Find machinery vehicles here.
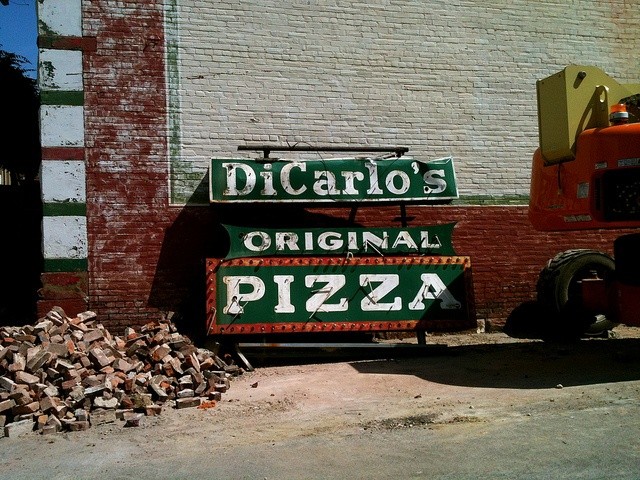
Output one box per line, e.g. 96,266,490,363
530,64,639,338
